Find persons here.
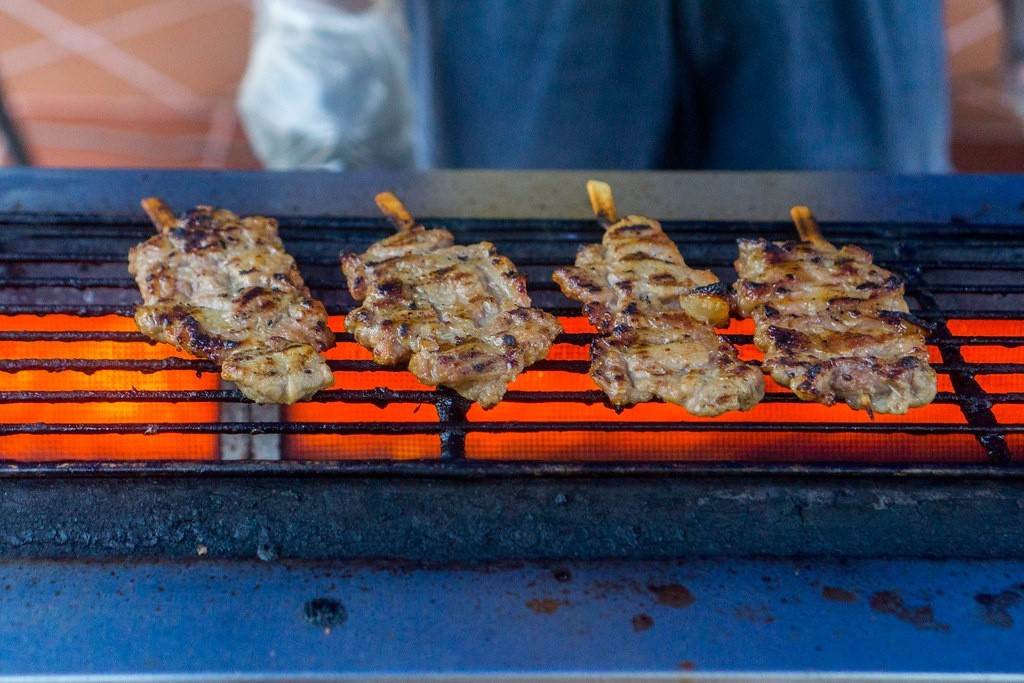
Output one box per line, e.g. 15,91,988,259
310,0,955,177
